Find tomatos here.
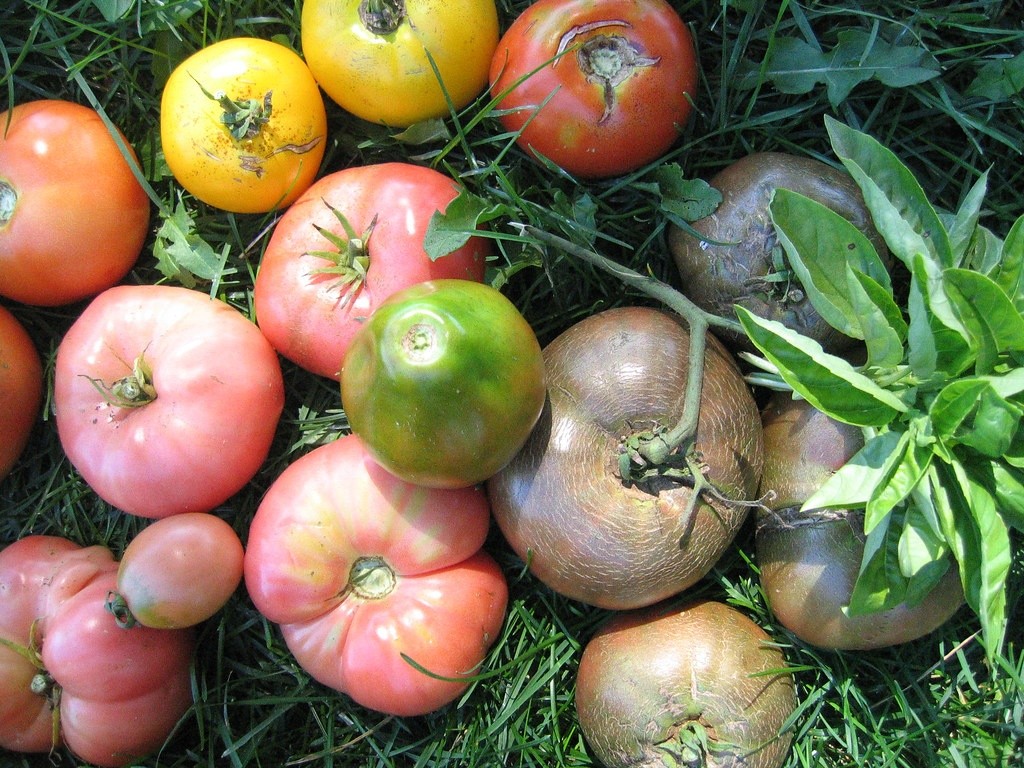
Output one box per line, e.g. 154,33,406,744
0,0,969,768
